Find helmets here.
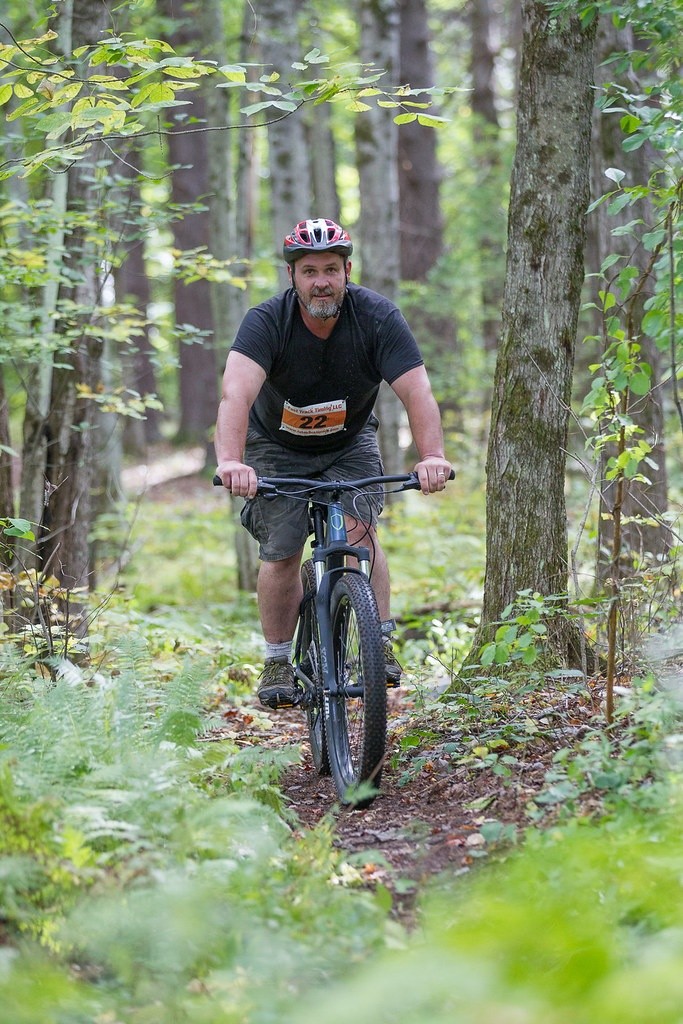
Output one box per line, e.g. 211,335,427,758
283,218,353,262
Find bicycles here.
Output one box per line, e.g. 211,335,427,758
211,463,457,809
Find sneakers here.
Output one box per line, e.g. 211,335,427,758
383,641,404,682
258,654,296,705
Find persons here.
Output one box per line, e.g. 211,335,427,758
212,218,451,706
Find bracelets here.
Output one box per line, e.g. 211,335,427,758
421,452,444,460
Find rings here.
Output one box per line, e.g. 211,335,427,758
438,473,444,475
